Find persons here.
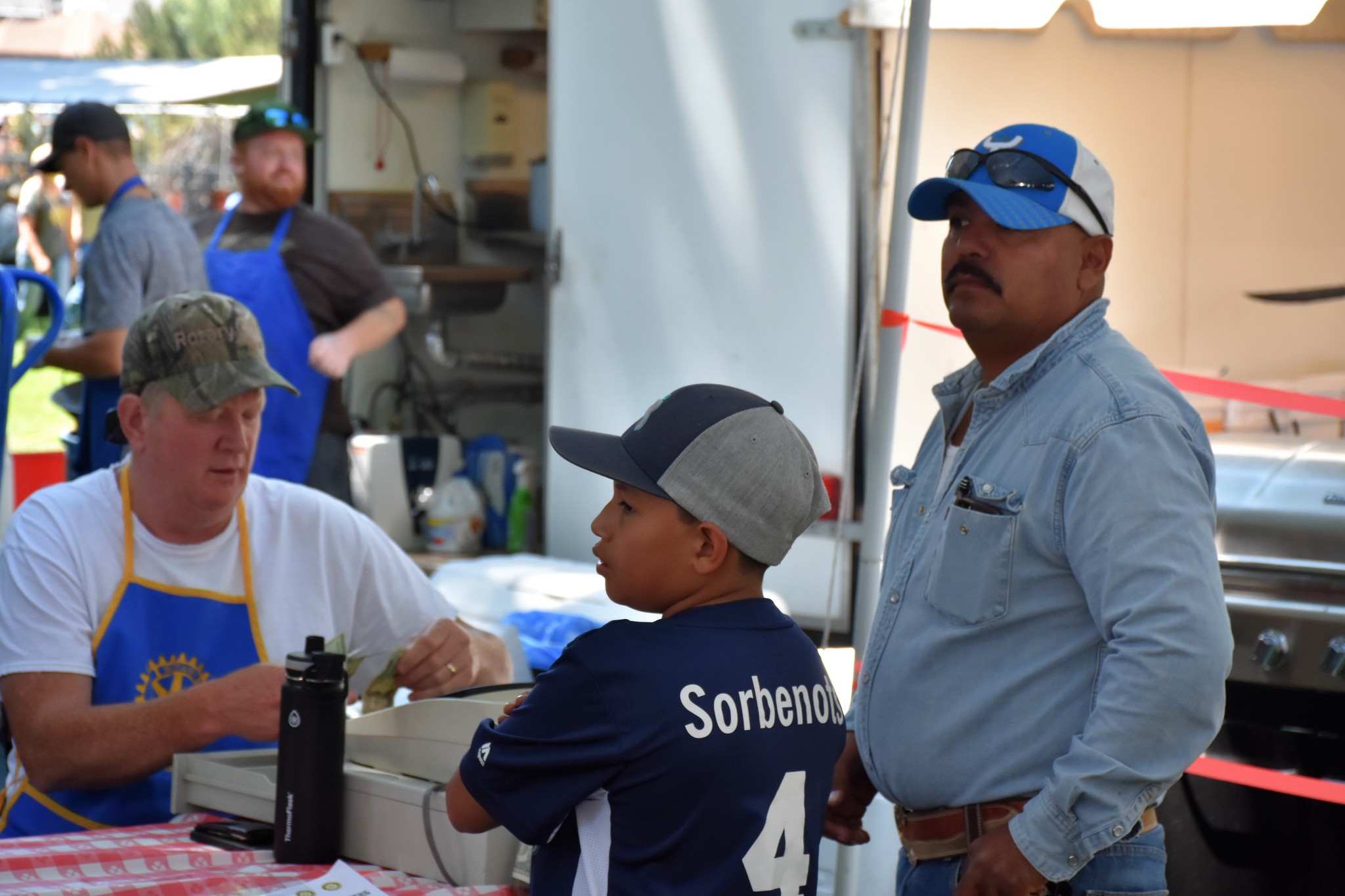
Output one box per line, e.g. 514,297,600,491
446,385,848,896
201,108,407,509
0,286,515,838
821,125,1236,896
31,100,212,479
14,171,82,333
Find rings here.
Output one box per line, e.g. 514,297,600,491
447,663,457,674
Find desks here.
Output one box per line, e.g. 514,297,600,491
0,812,529,896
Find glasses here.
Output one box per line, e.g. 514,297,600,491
233,108,309,130
946,148,1112,237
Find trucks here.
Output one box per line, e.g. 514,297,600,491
542,2,1345,848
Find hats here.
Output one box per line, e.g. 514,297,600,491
29,104,129,174
233,101,317,146
908,122,1114,239
549,383,831,566
121,292,298,414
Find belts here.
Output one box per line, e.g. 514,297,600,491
893,797,1159,865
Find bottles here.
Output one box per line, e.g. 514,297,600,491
272,636,347,862
424,434,534,554
